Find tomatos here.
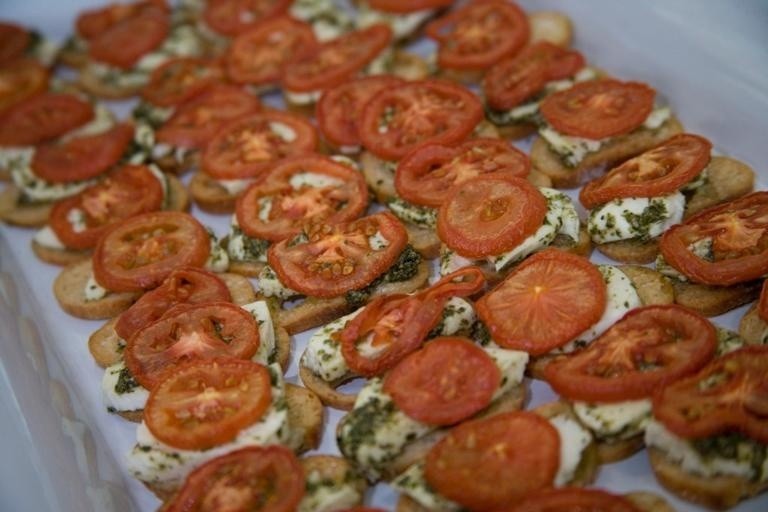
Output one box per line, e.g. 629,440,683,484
74,2,637,510
0,24,305,510
205,1,767,446
